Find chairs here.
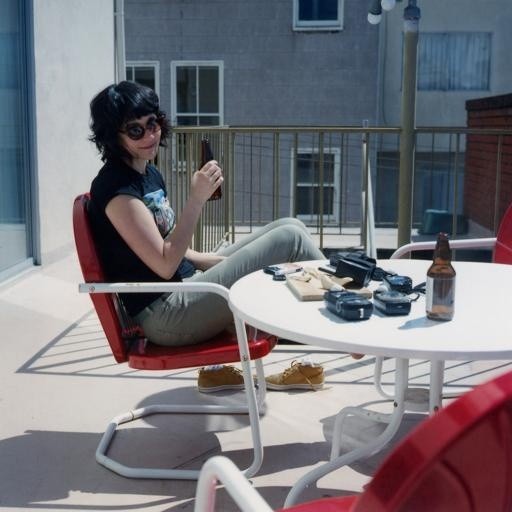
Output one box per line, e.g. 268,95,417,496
370,202,510,404
192,367,512,512
74,191,278,483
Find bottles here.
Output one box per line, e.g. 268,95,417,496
198,138,222,202
425,232,457,322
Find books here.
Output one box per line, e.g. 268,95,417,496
285,272,373,301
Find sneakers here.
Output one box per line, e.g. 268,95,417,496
264,359,325,392
198,365,259,393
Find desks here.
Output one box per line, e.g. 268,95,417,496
228,257,511,468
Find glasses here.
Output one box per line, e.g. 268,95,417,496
119,117,162,142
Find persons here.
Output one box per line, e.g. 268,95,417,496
85,82,328,347
